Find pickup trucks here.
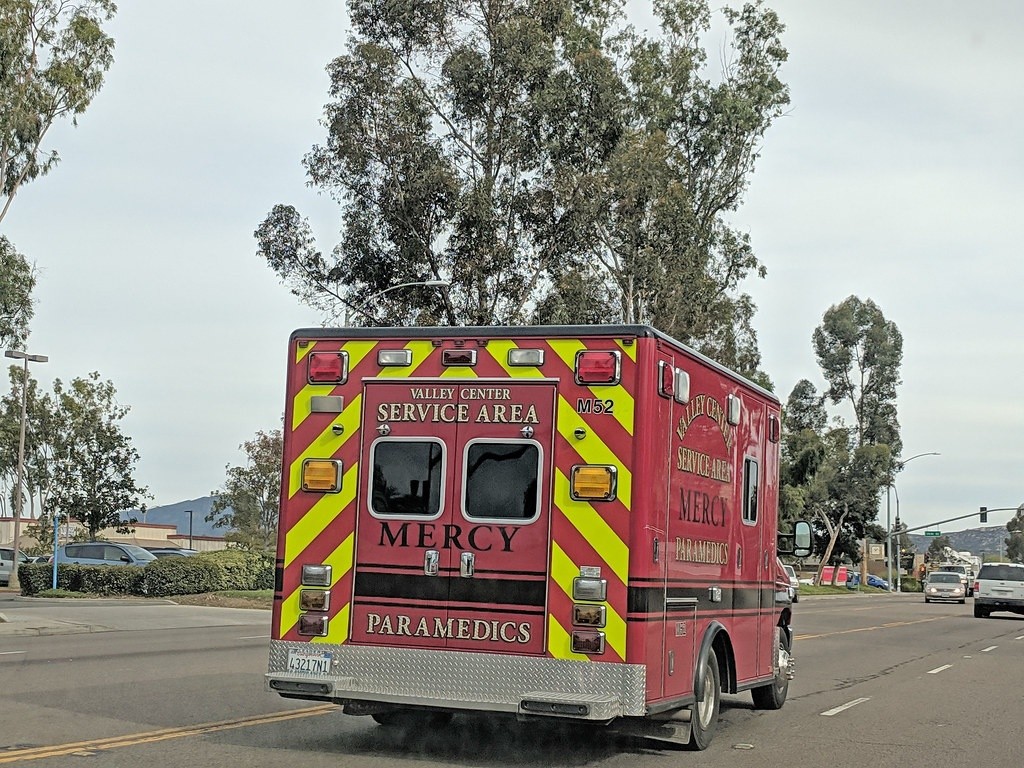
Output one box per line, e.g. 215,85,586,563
937,565,968,597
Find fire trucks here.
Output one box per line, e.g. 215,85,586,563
265,325,793,749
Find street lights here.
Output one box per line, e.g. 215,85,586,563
887,452,941,590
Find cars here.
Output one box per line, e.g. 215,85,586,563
921,572,966,603
851,574,894,590
783,565,799,602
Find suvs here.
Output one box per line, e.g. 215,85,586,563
57,541,159,567
973,563,1024,618
0,547,31,585
144,547,201,558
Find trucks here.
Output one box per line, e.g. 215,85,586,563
944,547,982,596
820,565,855,587
